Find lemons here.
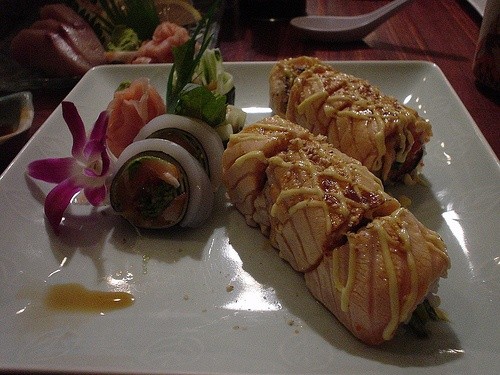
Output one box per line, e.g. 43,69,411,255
153,0,202,27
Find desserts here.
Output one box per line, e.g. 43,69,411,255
267,55,433,184
220,112,451,346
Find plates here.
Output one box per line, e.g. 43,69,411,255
0,60,500,375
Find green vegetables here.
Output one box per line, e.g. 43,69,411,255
164,12,228,127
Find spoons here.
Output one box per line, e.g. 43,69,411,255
291,0,411,41
